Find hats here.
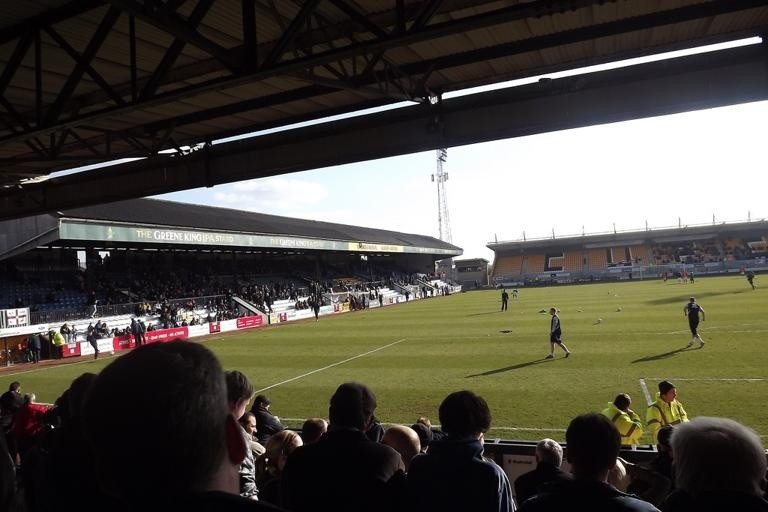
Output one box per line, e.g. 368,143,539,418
256,394,270,404
413,424,432,447
660,381,676,394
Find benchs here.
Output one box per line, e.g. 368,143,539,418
366,286,404,298
252,292,310,315
48,305,226,354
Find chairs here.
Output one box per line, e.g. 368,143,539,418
0,277,106,318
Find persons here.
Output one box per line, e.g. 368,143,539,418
683,296,708,347
739,264,757,290
0,256,480,364
494,282,520,312
0,341,767,512
545,307,571,358
660,267,695,286
